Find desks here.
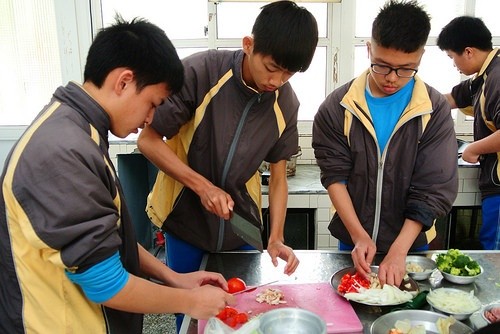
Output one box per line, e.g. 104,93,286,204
178,250,500,334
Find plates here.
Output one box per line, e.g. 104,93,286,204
330,266,418,306
370,309,475,334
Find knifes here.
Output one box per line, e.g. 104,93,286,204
229,281,279,296
230,209,263,253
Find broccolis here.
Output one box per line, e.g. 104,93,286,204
434,249,481,277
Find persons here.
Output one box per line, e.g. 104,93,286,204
0,11,239,334
441,16,500,250
310,0,460,290
137,0,319,334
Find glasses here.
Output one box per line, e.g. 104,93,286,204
369,46,421,78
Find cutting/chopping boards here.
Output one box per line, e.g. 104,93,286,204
198,282,364,334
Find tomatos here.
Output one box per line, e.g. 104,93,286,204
337,269,371,293
226,277,247,294
214,306,248,327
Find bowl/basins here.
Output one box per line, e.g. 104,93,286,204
440,265,484,284
256,308,327,334
406,255,437,280
426,288,481,320
482,302,500,325
